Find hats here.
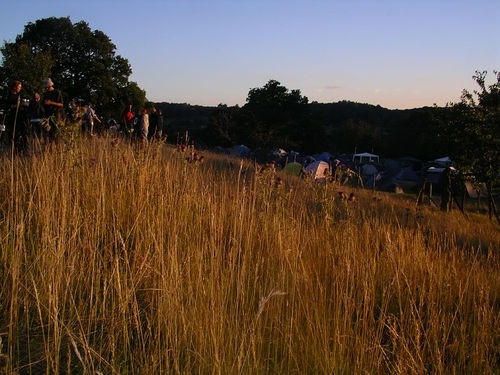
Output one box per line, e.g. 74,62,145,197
42,78,54,87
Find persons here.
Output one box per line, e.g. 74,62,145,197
41,78,63,147
64,95,203,164
437,168,451,213
29,92,41,132
1,80,27,153
453,170,466,216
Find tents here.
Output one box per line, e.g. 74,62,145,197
214,136,455,195
